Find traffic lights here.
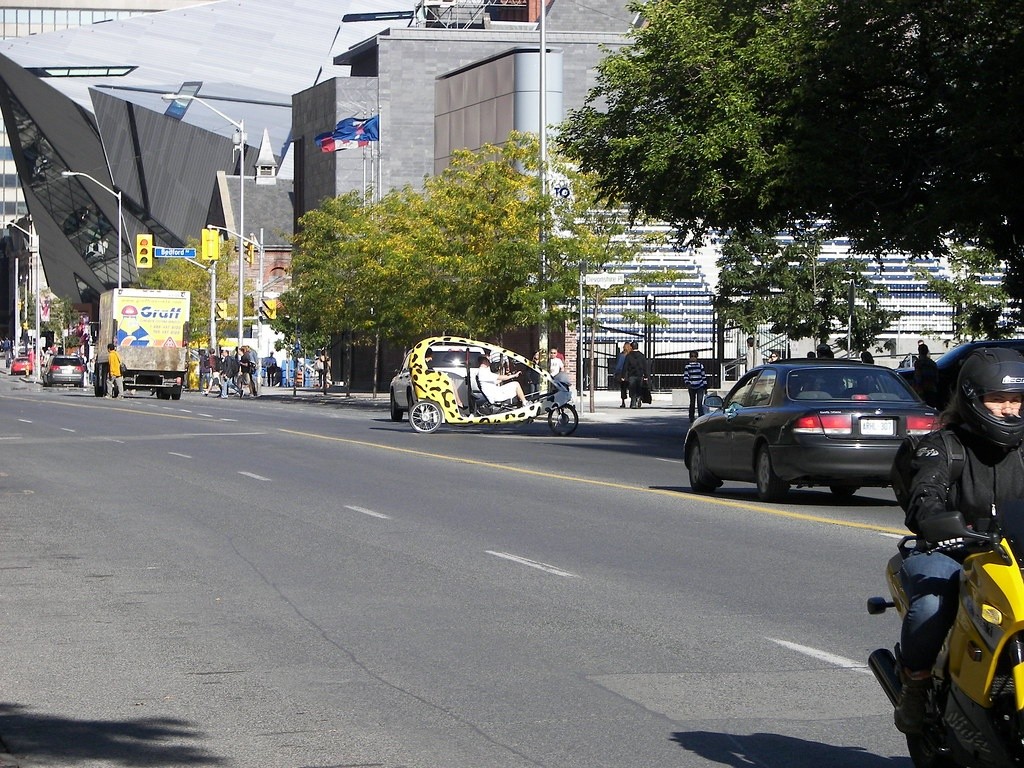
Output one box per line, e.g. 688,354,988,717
243,241,254,267
217,301,227,321
136,233,153,269
262,299,277,320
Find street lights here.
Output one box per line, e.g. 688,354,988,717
61,170,122,289
161,94,245,397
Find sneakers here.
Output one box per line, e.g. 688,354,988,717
895,670,935,733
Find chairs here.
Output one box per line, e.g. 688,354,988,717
476,375,513,410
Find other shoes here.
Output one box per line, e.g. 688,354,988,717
114,395,124,400
105,395,113,400
619,401,641,409
239,390,243,399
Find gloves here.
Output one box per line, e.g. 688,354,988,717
938,536,964,553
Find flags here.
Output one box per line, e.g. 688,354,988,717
333,114,379,140
315,130,369,152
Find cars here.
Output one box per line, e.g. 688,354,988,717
388,344,485,422
683,357,953,503
41,354,87,388
10,356,32,375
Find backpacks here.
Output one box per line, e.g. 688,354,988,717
226,356,239,374
890,430,966,516
213,355,222,372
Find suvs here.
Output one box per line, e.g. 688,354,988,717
894,337,1024,412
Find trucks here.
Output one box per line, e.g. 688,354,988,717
90,287,191,400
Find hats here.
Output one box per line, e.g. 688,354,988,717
818,343,830,351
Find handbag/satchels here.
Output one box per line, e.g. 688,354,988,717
246,360,256,374
120,364,127,374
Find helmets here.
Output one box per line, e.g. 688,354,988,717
954,347,1024,450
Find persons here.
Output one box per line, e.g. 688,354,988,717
488,340,510,374
103,344,124,399
861,352,874,392
892,348,1024,736
477,355,529,406
614,341,649,408
807,344,834,358
765,352,779,394
311,354,324,386
914,344,938,406
684,351,707,422
4,338,10,352
82,354,95,386
527,350,539,397
265,352,277,387
550,348,564,377
45,347,53,354
188,344,261,398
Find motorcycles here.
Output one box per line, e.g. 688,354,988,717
865,432,1024,768
